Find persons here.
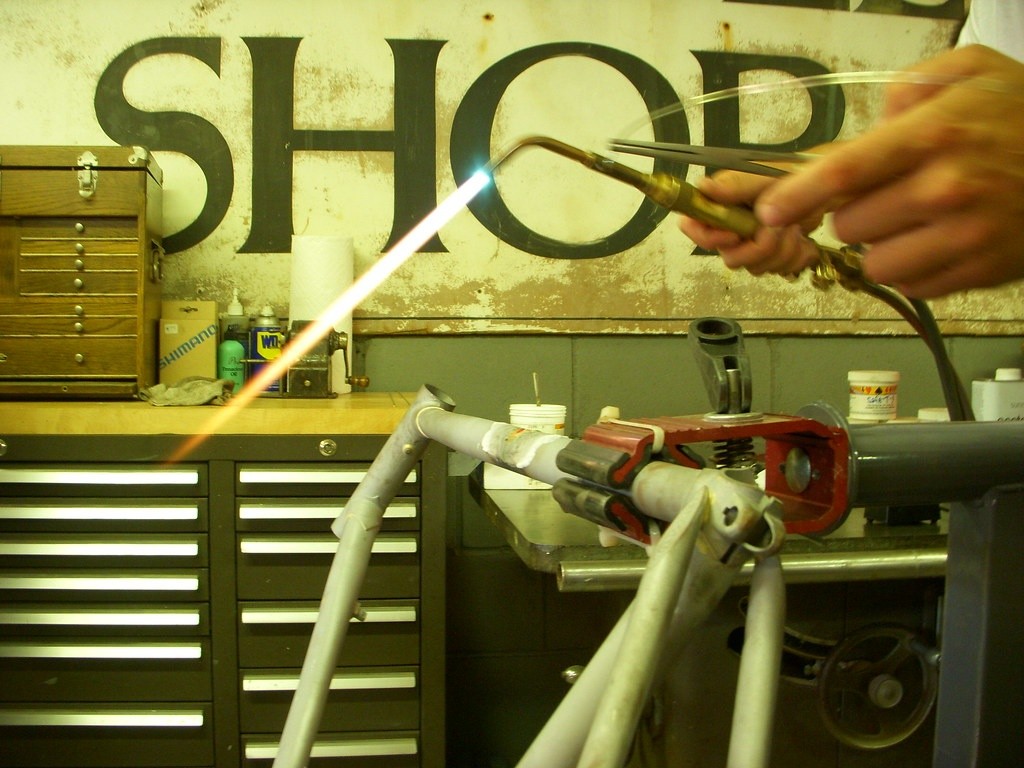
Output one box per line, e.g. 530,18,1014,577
680,0,1024,299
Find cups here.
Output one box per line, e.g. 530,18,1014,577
509,403,567,435
847,370,900,420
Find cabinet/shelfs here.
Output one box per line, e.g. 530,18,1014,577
1,393,449,766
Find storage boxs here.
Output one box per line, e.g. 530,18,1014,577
1,143,164,399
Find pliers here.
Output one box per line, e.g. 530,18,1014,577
609,138,828,179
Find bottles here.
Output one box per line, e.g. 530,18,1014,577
219,287,282,398
